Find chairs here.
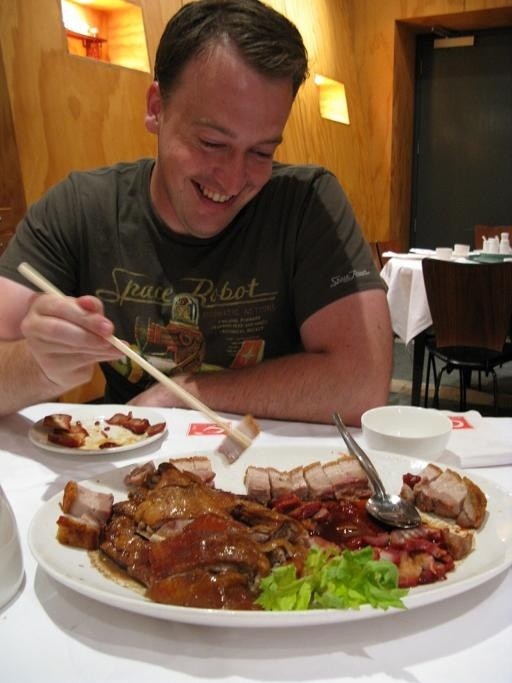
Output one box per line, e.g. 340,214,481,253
369,238,510,413
469,221,512,250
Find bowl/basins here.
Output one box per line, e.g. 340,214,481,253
360,405,453,461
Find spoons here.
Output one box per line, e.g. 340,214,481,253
327,409,423,531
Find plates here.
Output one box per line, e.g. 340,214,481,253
32,405,170,458
34,439,510,631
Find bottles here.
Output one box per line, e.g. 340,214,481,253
412,232,509,261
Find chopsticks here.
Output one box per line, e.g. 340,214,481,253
11,261,253,448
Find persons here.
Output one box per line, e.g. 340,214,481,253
0,0,393,428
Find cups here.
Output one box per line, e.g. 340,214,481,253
1,486,28,609
